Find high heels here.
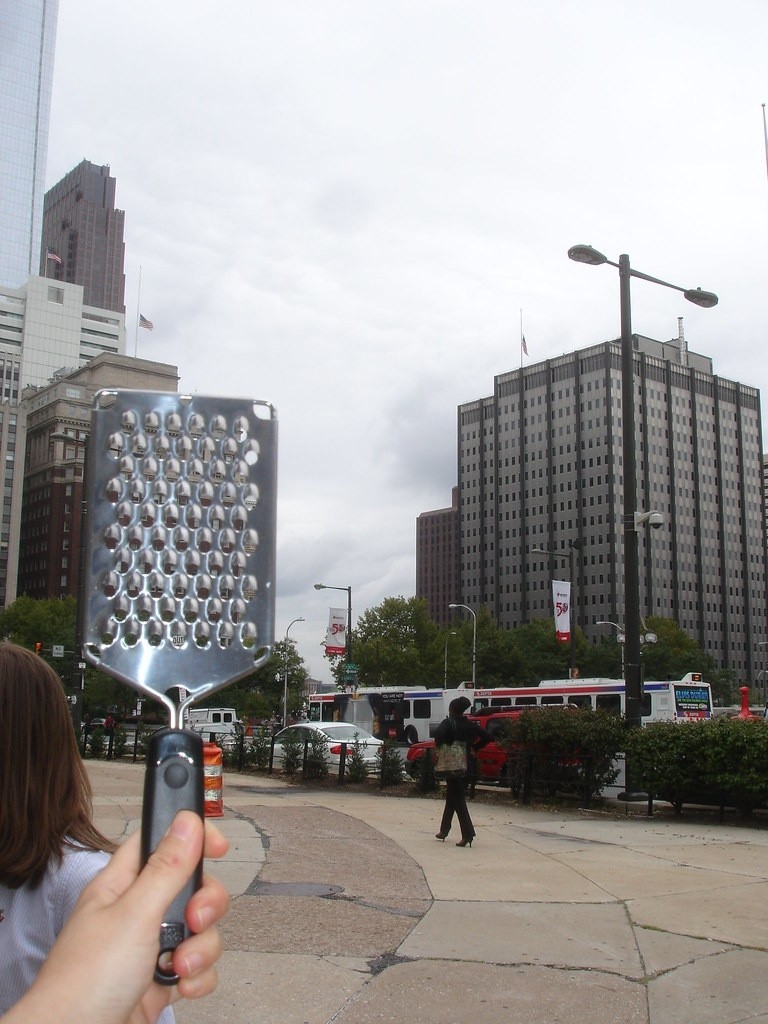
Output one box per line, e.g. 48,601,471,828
436,832,446,842
455,838,474,848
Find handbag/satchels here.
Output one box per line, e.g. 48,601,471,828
434,716,467,775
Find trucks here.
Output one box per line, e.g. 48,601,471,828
185,707,237,745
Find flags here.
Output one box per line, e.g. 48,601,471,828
522,336,528,354
138,313,152,329
47,248,62,264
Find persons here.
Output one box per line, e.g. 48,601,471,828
104,713,113,735
0,811,228,1024
436,697,491,847
0,641,176,1024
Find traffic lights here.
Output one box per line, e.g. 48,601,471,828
35,642,41,654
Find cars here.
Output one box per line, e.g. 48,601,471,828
82,717,119,735
251,721,384,775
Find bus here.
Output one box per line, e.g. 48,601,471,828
402,672,715,758
307,686,427,744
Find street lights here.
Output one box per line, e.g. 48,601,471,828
314,584,351,685
596,621,624,678
284,617,305,727
531,549,574,677
444,632,456,688
568,243,718,801
449,603,476,687
617,632,657,701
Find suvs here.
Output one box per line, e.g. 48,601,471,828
406,705,581,787
